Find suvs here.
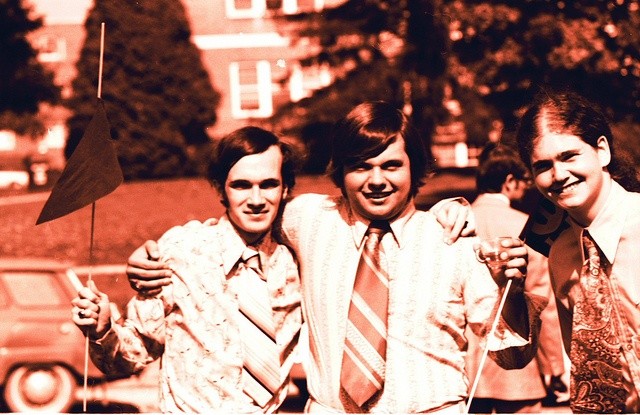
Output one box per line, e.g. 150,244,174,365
2,258,104,414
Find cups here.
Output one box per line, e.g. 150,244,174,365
475,235,515,268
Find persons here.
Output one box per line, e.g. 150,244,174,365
125,100,550,413
70,126,478,413
497,91,640,413
462,141,568,414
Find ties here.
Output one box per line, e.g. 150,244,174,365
239,245,282,408
340,221,388,407
569,234,625,414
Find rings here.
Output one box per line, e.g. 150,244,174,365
78,309,84,318
